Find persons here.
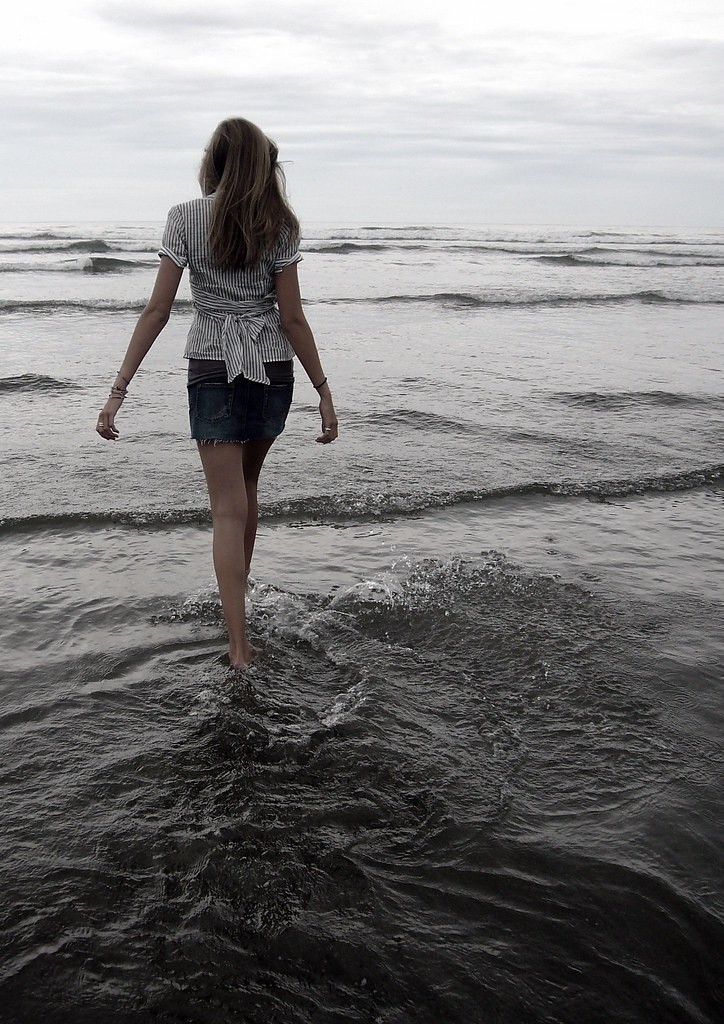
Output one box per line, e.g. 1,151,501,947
92,114,339,670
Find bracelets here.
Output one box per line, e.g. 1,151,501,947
109,387,128,399
116,370,130,385
312,377,328,389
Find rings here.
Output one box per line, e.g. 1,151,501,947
99,422,104,427
325,427,332,431
